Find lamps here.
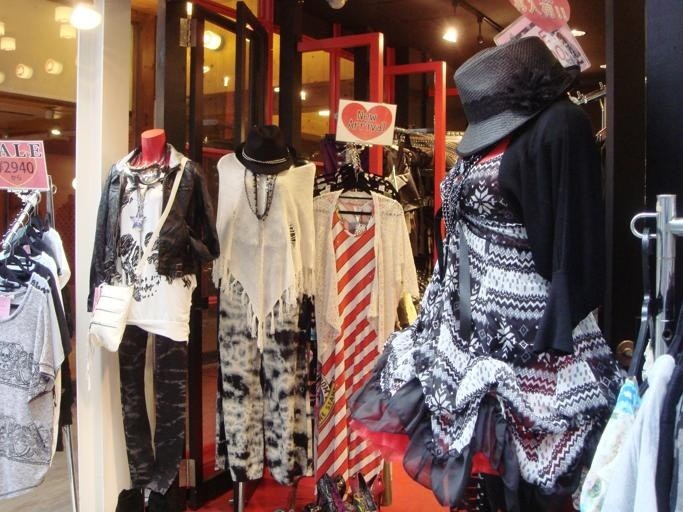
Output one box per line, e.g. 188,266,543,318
476,13,487,49
442,1,460,43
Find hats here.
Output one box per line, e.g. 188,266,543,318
234,122,294,174
453,37,581,157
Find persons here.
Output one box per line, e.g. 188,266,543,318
425,37,606,512
98,127,218,493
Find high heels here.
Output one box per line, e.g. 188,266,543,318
303,473,386,511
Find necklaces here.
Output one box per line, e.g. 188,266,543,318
130,180,151,227
240,166,288,222
440,155,504,237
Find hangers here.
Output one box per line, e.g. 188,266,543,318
0,191,62,302
620,231,683,434
314,142,406,218
402,134,468,165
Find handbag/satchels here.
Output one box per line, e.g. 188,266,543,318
87,283,134,353
